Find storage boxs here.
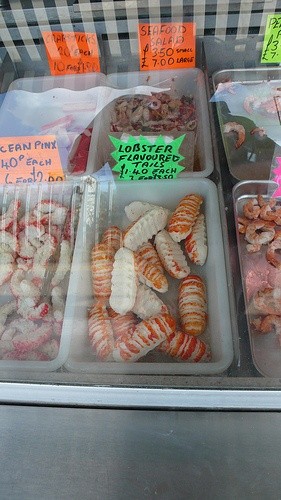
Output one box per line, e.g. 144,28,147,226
85,66,215,179
0,181,87,375
63,177,233,373
0,73,107,181
